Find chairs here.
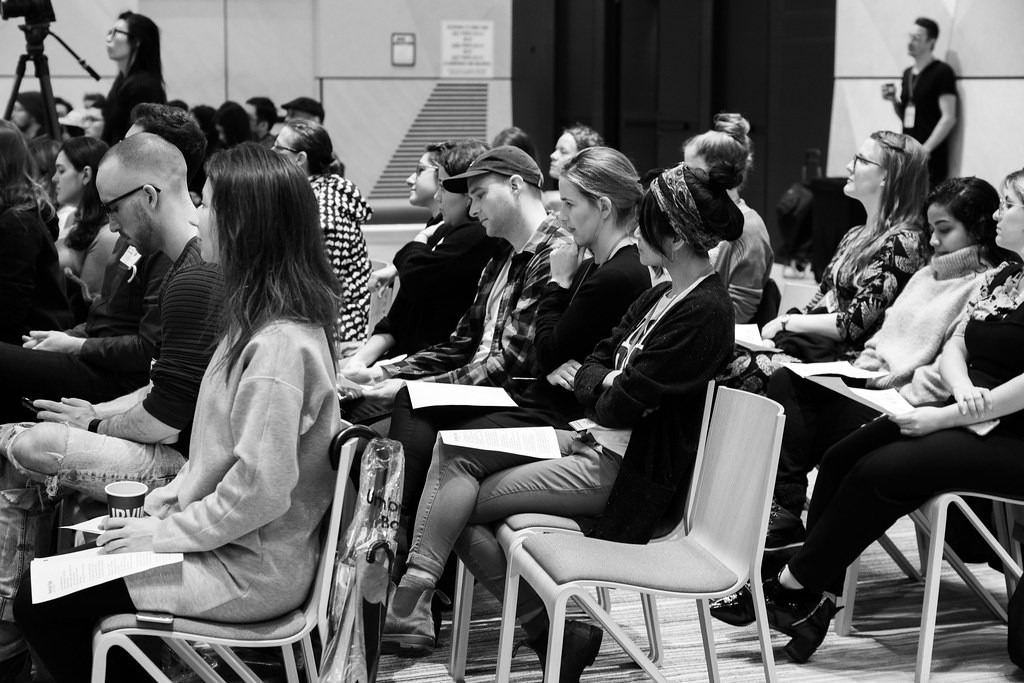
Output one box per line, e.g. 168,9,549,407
450,378,786,683
366,260,393,341
91,420,358,683
804,462,1024,683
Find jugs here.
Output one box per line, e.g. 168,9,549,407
801,146,823,185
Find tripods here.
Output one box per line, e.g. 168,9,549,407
4,23,100,142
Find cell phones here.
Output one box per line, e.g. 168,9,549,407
22,398,47,412
136,610,174,624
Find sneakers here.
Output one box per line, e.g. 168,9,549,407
764,502,806,551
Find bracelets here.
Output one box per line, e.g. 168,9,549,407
88,419,102,433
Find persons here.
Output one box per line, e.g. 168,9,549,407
1,92,375,423
100,9,166,148
881,18,960,195
703,167,1024,664
1,142,341,683
0,132,233,619
335,113,1024,683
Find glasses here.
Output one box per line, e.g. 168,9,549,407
416,163,440,174
108,28,129,38
101,184,161,219
271,140,298,152
999,200,1024,212
853,154,887,170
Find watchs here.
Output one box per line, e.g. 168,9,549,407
781,314,791,330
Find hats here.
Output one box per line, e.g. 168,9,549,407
58,108,83,128
443,145,544,194
282,97,324,124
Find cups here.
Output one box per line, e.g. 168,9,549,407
105,481,149,551
882,83,895,96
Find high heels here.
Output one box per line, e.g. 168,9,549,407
745,582,845,663
709,567,810,627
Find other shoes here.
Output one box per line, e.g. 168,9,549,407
381,586,452,659
513,619,602,683
